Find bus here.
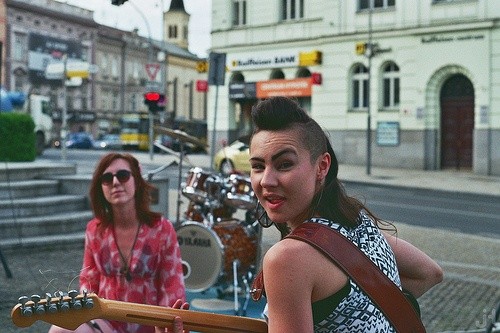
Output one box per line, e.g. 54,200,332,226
113,110,161,154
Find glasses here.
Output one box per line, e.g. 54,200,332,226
100,169,132,187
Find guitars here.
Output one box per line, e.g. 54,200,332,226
11,285,421,333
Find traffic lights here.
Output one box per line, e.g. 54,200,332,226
144,89,161,104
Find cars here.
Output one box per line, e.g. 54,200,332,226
62,118,211,156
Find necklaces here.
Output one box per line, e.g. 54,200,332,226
114,219,142,281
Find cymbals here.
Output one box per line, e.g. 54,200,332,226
152,141,195,168
150,125,210,147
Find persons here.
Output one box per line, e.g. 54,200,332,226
49,154,187,333
154,96,444,333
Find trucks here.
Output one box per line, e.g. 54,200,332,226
0,88,58,154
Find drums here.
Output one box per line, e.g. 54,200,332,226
182,167,222,209
184,197,231,223
218,174,258,210
174,220,260,291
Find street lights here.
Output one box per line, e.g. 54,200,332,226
111,0,154,158
